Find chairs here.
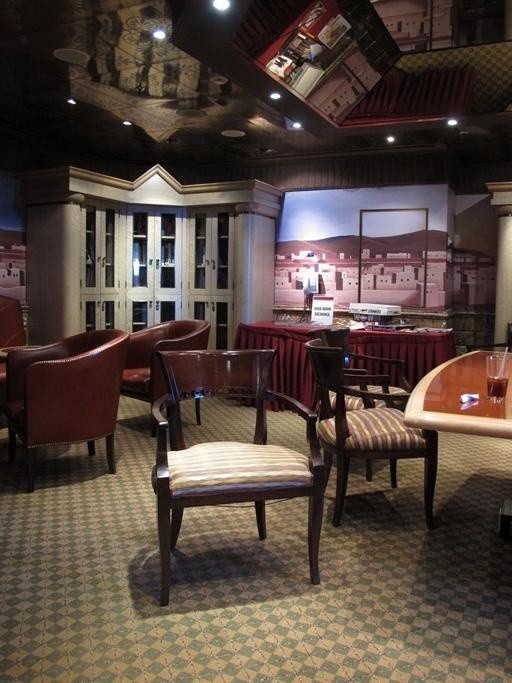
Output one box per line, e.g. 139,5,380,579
121,320,211,438
151,349,327,606
304,327,439,531
0,296,129,492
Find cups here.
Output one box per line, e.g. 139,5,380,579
484,352,510,405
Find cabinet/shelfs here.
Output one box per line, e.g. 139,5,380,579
15,163,285,349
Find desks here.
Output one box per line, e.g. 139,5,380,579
234,319,453,413
401,348,511,537
